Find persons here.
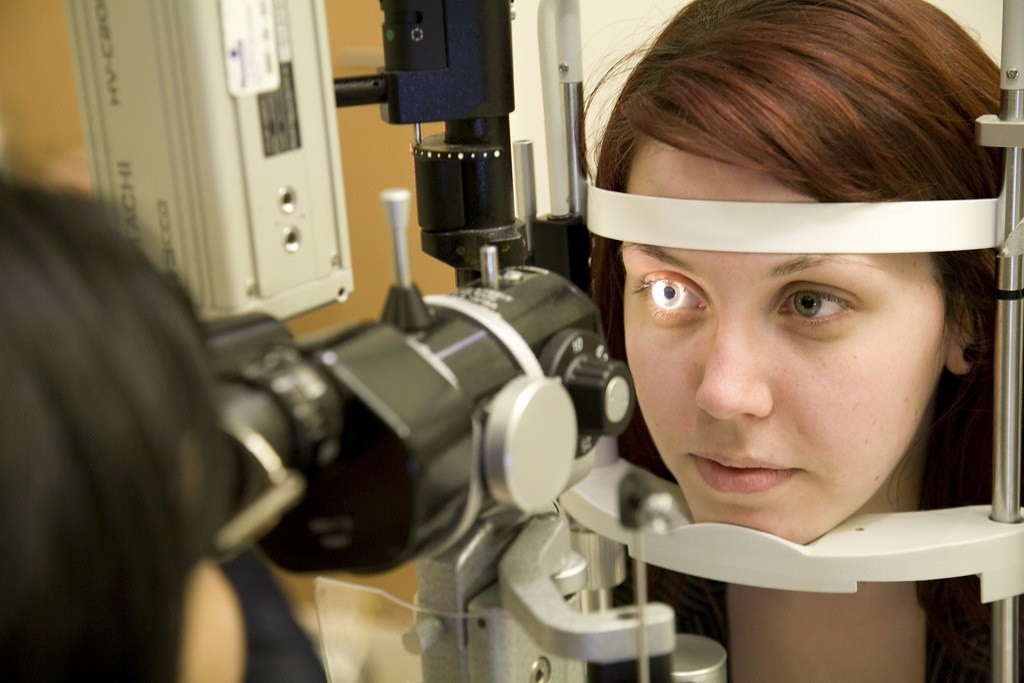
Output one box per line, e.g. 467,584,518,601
0,181,247,683
586,1,1021,683
